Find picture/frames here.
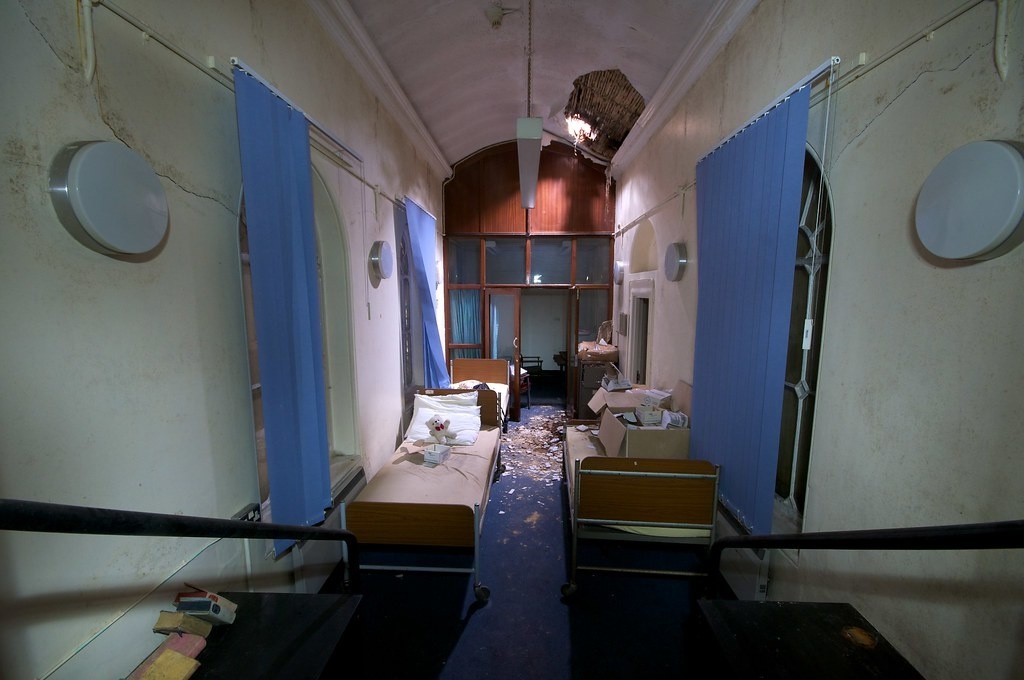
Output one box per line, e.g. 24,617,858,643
619,313,627,336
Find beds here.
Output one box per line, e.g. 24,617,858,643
561,419,720,598
450,359,509,433
340,389,502,604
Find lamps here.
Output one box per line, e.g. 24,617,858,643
370,241,393,279
47,139,169,260
517,118,540,208
664,244,687,281
614,262,624,285
914,138,1024,263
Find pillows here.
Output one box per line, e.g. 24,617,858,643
405,407,481,445
404,391,478,439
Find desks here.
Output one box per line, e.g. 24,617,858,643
125,593,360,680
554,351,567,374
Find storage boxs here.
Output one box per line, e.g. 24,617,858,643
579,341,618,362
423,445,450,464
588,384,656,421
598,392,690,457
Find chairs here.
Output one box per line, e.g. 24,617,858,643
498,356,531,410
520,353,542,376
